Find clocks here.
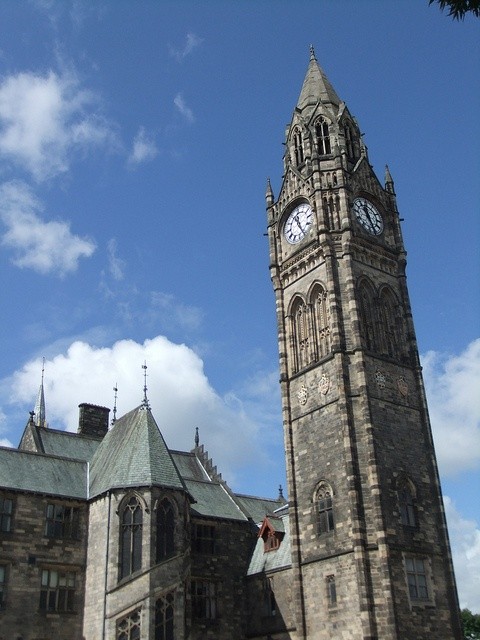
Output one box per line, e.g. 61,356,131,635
277,195,313,246
353,192,391,239
